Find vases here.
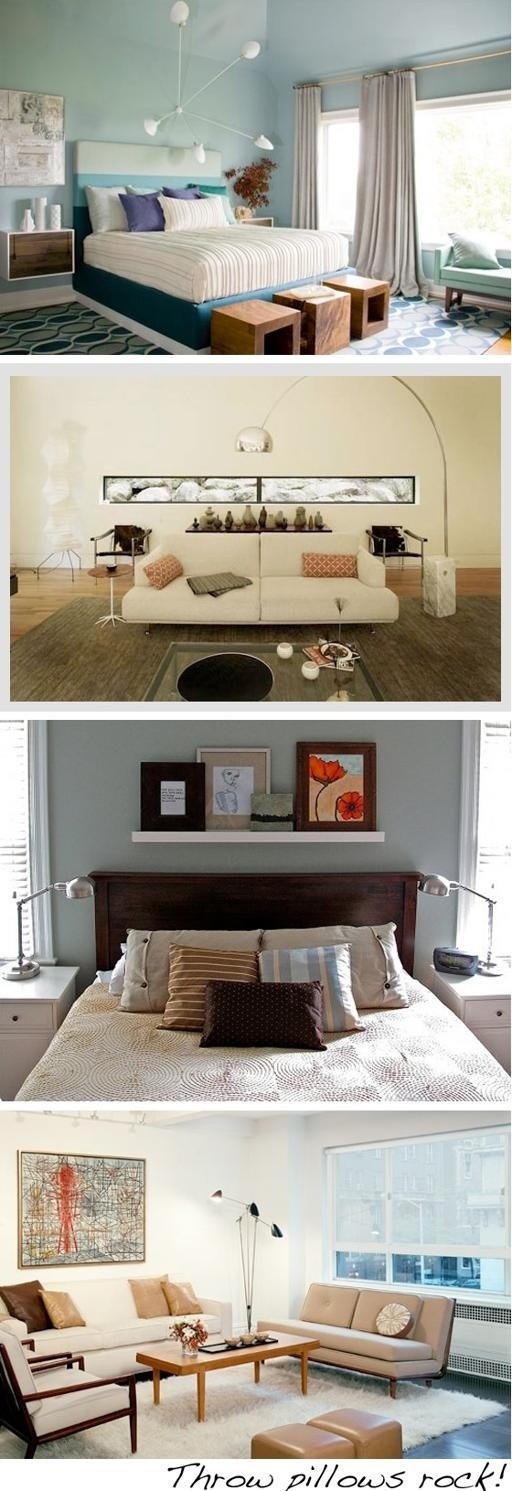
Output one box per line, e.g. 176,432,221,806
181,1340,199,1357
18,208,38,232
34,196,48,230
47,203,63,230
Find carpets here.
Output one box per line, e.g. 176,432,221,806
11,591,500,701
0,1356,509,1461
0,294,511,356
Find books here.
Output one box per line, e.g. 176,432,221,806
304,638,361,672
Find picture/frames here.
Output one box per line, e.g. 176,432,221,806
196,746,270,832
294,739,377,832
16,1146,149,1270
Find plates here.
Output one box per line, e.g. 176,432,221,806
320,642,354,662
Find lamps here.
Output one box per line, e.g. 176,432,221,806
209,1188,287,1334
135,1,285,163
230,374,455,560
0,874,99,985
413,871,509,980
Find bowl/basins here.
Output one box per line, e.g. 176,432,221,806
224,1338,241,1349
106,564,118,572
254,1333,270,1342
239,1334,256,1346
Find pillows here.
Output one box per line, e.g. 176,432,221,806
37,1288,86,1329
127,1274,179,1320
157,1279,204,1320
0,1279,56,1336
82,180,238,237
107,918,417,1055
142,550,186,592
370,1301,416,1339
299,550,361,580
445,229,504,272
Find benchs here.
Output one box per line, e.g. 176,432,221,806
432,242,510,319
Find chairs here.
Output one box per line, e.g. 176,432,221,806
364,524,430,583
88,525,154,585
0,1329,143,1460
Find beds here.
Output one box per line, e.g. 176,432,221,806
138,639,391,701
11,867,510,1103
69,138,360,355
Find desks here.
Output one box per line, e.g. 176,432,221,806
87,562,135,630
31,541,84,586
184,522,335,535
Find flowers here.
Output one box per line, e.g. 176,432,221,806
307,753,368,823
167,1319,211,1353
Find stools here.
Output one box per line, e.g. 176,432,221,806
322,274,390,340
205,298,305,355
248,1425,356,1461
271,283,354,355
307,1409,409,1459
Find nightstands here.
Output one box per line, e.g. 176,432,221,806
236,216,274,229
0,961,82,1101
424,958,510,1079
1,227,78,286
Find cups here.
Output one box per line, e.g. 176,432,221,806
48,205,61,230
277,642,294,660
35,198,48,231
302,662,320,681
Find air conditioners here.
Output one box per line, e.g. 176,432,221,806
444,1298,511,1383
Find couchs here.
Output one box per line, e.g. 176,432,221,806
119,532,401,638
0,1275,238,1381
251,1281,460,1402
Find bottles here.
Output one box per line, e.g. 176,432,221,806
21,208,35,232
192,506,325,532
